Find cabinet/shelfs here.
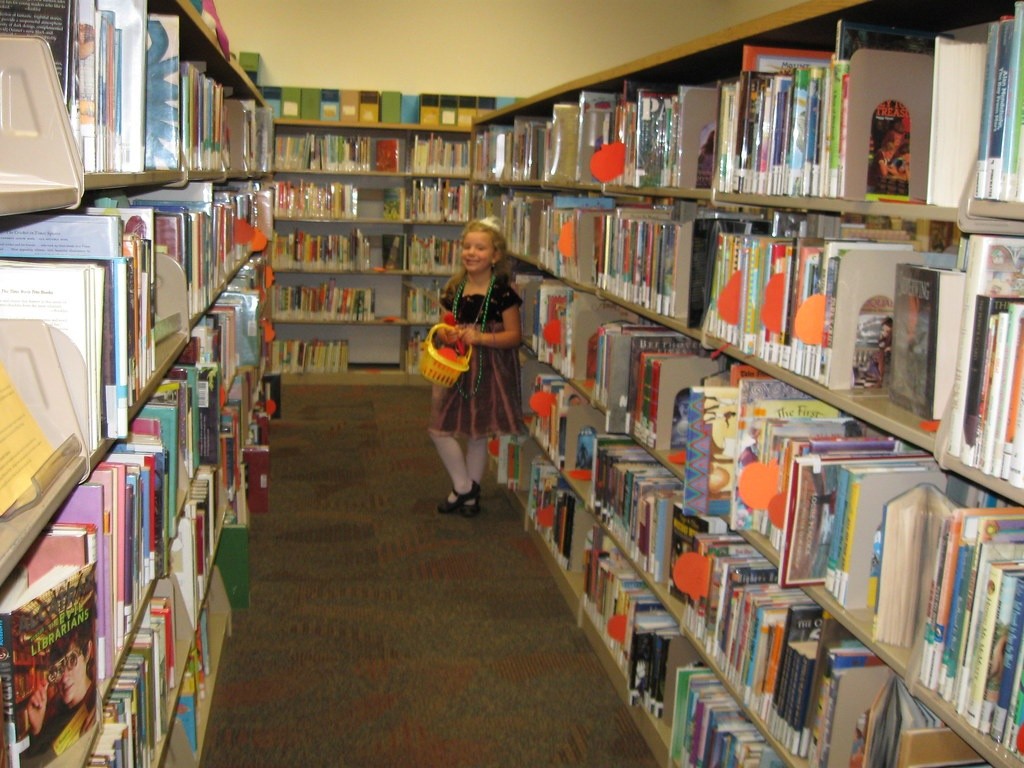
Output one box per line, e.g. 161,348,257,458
469,0,1024,768
265,116,473,387
0,0,276,768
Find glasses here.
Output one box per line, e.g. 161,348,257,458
47,650,78,684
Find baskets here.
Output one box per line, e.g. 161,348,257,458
421,323,473,387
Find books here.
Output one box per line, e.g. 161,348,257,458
0,0,271,209
465,0,1024,214
270,132,477,373
510,268,1023,768
2,188,271,520
476,185,1024,491
2,258,283,768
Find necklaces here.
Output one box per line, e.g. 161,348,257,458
450,273,496,399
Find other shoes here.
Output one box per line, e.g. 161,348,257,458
462,496,480,516
437,480,481,514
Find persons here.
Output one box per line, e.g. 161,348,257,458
428,219,524,516
